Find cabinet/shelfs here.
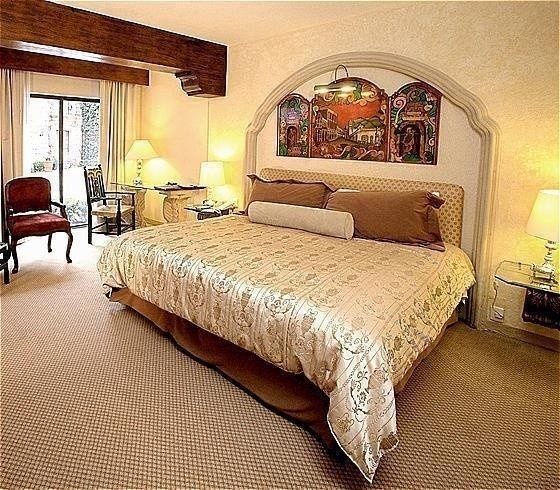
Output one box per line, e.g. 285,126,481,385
184,202,235,221
495,260,559,329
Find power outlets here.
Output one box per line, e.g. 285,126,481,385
489,305,504,322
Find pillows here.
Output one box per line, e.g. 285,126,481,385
237,174,336,224
247,201,355,241
325,188,447,253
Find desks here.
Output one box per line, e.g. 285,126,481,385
110,181,158,229
154,185,205,223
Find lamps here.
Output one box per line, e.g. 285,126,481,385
316,64,358,97
198,161,226,204
524,190,559,281
125,139,160,183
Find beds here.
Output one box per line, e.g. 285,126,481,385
97,167,474,484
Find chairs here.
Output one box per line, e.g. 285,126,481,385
4,176,73,275
84,162,137,245
